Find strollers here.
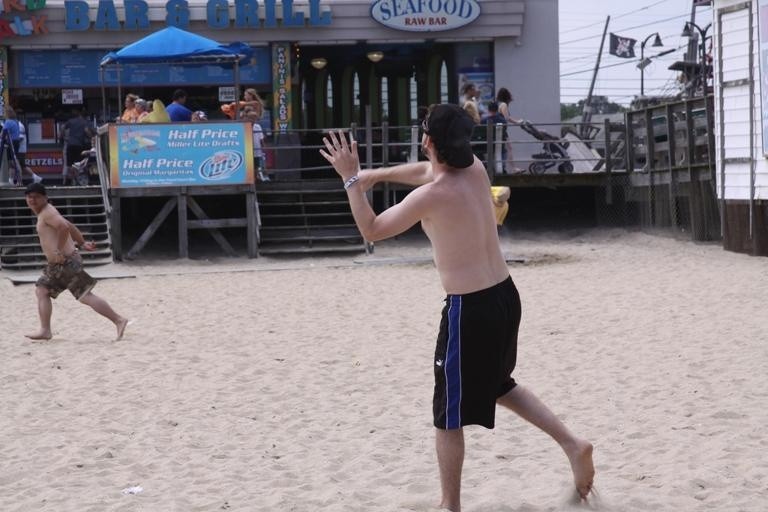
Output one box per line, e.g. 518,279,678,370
520,120,573,173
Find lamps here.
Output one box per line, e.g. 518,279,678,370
309,46,329,70
365,46,385,64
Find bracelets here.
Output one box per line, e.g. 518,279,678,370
79,242,85,246
343,175,359,190
56,250,63,255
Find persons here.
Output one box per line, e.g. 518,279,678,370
319,103,594,512
61,124,77,185
458,83,524,175
15,108,43,183
61,106,93,164
24,182,128,340
0,106,23,188
116,87,270,182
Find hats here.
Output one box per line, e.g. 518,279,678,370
24,182,45,194
432,103,474,168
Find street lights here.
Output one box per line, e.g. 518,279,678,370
640,32,664,95
681,21,712,96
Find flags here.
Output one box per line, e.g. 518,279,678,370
610,32,636,57
